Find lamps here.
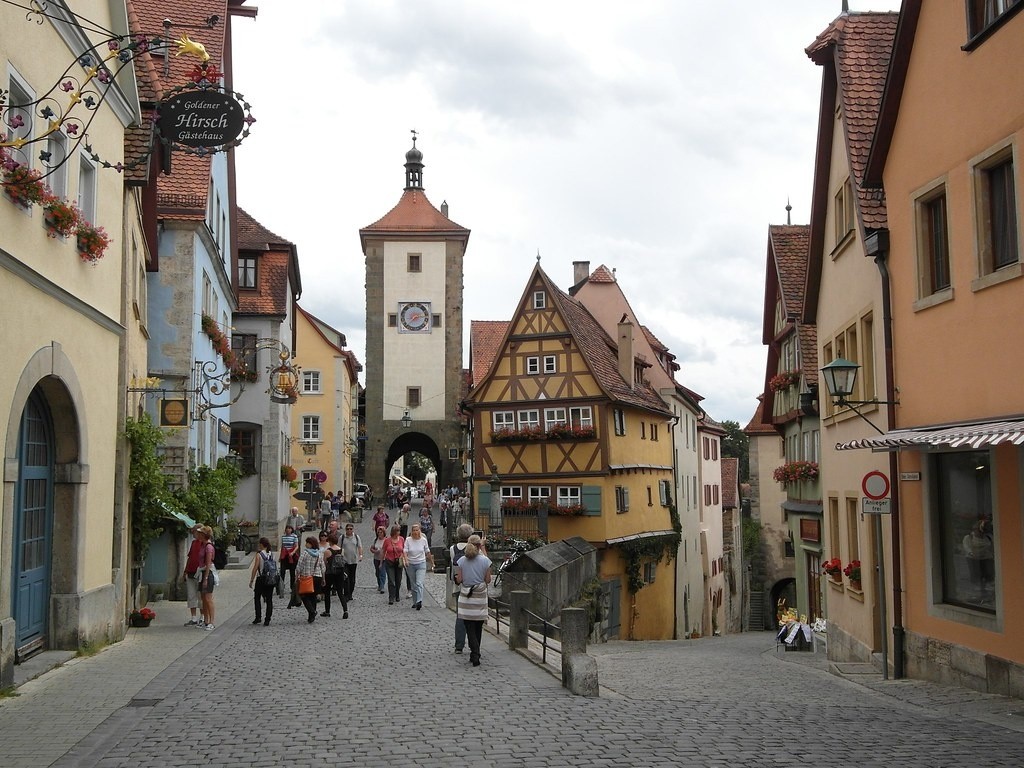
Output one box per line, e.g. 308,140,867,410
820,349,901,407
400,407,412,428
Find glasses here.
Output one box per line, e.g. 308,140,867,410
345,528,353,531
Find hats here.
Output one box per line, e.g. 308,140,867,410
376,505,385,510
192,522,204,529
196,526,213,535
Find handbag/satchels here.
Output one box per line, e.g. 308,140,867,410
298,576,314,594
396,557,405,568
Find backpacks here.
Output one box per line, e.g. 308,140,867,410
260,552,278,585
451,544,468,581
331,553,344,569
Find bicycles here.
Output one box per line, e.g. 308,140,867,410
235,532,252,556
494,539,530,587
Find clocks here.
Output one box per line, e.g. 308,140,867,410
398,302,432,334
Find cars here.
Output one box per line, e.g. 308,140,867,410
403,487,418,499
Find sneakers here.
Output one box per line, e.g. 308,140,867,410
195,620,205,629
195,622,209,629
184,620,198,627
204,624,216,631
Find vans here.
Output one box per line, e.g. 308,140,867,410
354,484,371,506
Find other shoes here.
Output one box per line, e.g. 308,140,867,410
252,618,262,624
395,596,401,602
343,612,348,619
454,649,463,654
380,588,385,594
412,603,416,609
308,611,318,623
405,590,412,599
320,612,331,618
388,600,394,605
469,653,481,667
415,603,423,611
263,621,270,626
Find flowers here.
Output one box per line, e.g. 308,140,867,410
821,558,842,574
201,309,259,383
845,559,861,580
0,163,113,267
129,608,156,620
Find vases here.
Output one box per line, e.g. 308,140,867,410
833,572,842,581
132,619,150,627
850,579,861,590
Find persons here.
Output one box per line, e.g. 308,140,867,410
278,525,299,591
373,506,389,533
184,523,219,630
285,506,305,556
402,524,435,610
248,537,275,625
384,483,411,511
418,478,437,547
364,485,373,510
370,526,387,593
317,489,364,532
455,534,492,666
294,521,362,623
449,523,488,654
395,504,410,540
437,484,471,528
379,524,405,604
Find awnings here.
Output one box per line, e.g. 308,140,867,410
401,476,413,484
396,477,407,484
836,420,1024,452
